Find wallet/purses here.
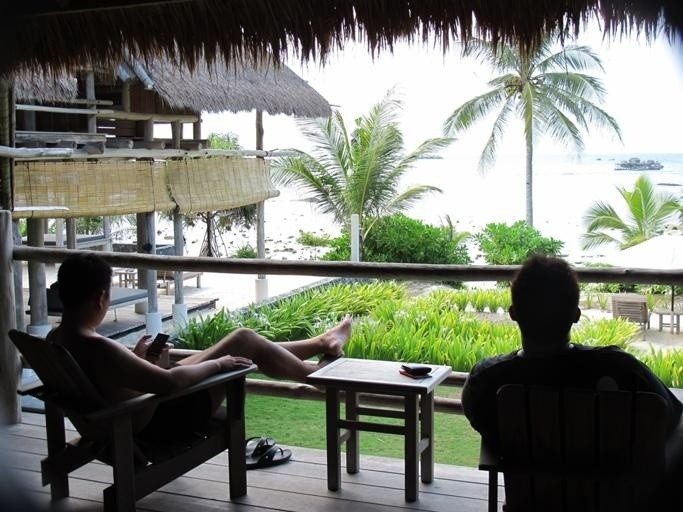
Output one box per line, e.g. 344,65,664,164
403,363,431,375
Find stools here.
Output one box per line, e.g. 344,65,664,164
304,356,452,503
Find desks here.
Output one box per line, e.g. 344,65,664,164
654,311,682,334
114,271,137,289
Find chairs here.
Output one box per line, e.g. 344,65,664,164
6,327,258,511
478,383,672,512
611,294,651,341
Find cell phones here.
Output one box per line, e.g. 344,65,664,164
146,332,170,357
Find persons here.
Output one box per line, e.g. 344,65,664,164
461,256,681,512
43,252,352,456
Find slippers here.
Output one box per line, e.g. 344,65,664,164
247,444,292,470
245,437,275,457
